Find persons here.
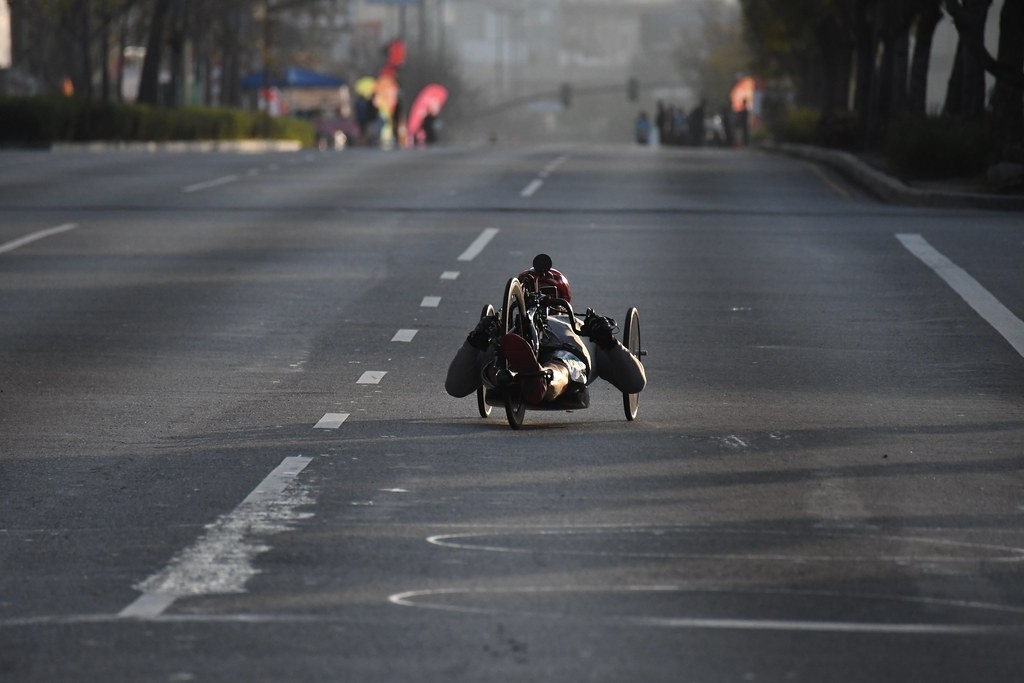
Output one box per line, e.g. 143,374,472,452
633,96,749,149
445,265,648,403
320,87,443,145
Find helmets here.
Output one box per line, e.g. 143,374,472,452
517,265,571,302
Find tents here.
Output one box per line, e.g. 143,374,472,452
241,63,348,115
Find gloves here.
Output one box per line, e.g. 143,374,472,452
580,311,618,349
467,316,502,350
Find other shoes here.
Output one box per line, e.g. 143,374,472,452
503,333,548,404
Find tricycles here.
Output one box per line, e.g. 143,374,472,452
476,254,649,431
